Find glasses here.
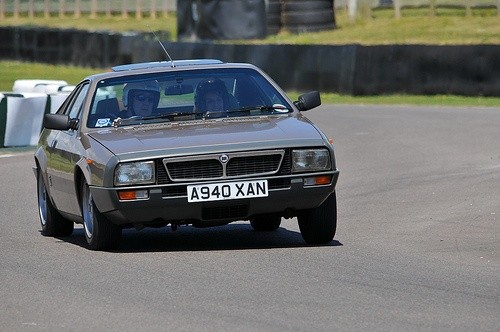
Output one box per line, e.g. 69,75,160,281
132,93,156,103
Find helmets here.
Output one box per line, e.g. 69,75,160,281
122,79,161,107
194,80,230,114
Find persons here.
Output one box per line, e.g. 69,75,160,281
122,80,161,117
194,80,230,117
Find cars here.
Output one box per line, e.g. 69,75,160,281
34,58,337,250
